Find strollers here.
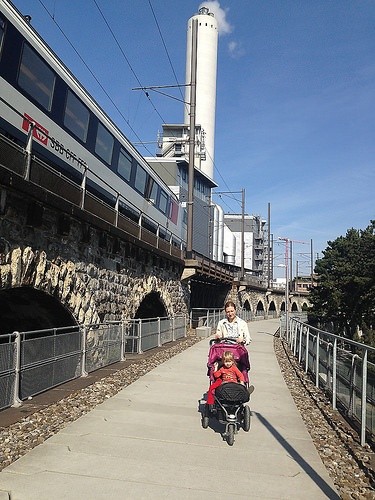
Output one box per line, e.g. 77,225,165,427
203,339,250,446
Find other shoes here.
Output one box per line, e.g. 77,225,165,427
207,405,217,415
247,385,254,394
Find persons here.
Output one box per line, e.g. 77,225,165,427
215,302,252,346
213,351,254,395
